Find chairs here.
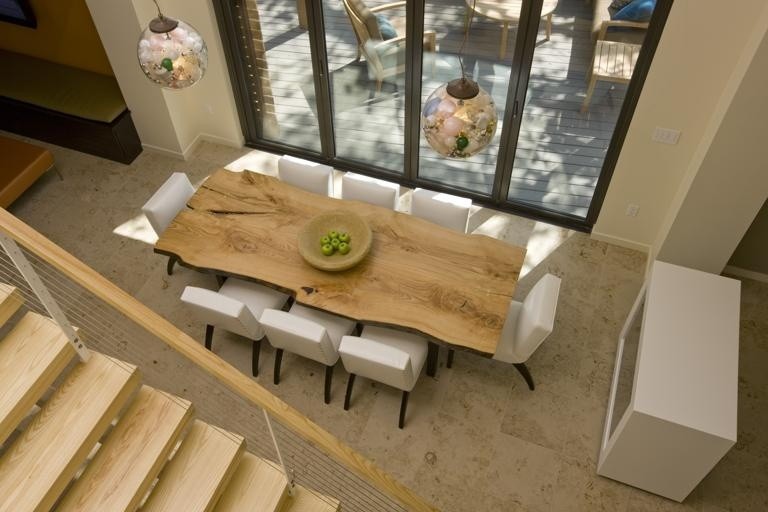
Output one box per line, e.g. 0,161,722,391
337,326,429,430
591,0,656,40
278,154,334,197
343,0,435,93
411,188,472,233
493,273,562,390
180,277,293,377
580,21,649,114
141,173,195,275
259,300,363,403
342,172,399,209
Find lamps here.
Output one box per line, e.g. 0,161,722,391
422,0,498,158
137,1,207,90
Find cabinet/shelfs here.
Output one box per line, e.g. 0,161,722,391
0,47,143,165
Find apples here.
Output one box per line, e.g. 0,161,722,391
320,230,351,255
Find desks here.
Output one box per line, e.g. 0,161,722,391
464,0,558,59
597,260,741,504
154,167,528,378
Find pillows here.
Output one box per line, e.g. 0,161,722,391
608,0,632,17
376,14,397,41
613,0,656,21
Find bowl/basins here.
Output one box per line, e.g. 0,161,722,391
297,207,373,272
